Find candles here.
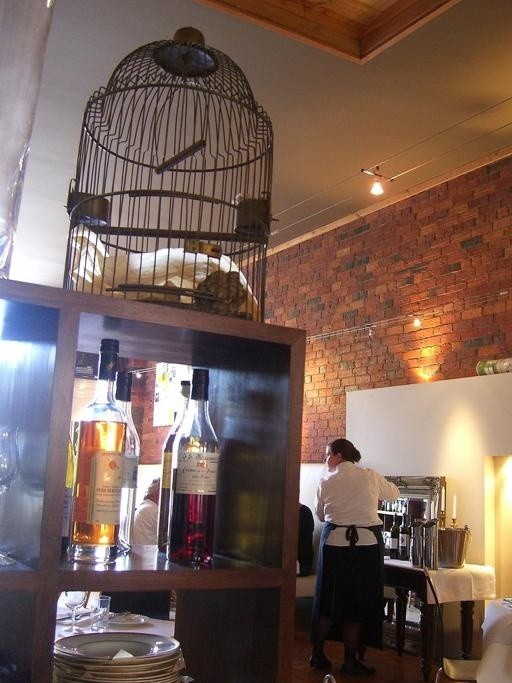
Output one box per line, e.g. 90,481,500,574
452,494,456,518
440,486,446,511
430,501,434,519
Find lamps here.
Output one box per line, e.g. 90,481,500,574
359,164,394,197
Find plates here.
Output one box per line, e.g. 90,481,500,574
53,631,186,682
56,606,152,625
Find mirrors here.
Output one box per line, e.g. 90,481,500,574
378,475,447,659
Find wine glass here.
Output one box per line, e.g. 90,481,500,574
1,425,22,567
64,590,87,636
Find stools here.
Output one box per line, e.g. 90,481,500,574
296,574,317,598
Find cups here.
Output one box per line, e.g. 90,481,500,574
90,595,111,633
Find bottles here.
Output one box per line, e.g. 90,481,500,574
390,512,410,560
69,338,221,566
377,499,406,511
413,519,437,570
475,358,511,375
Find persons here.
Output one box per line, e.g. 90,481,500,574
306,439,401,677
131,476,161,545
297,501,315,578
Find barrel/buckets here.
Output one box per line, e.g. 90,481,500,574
437,527,472,569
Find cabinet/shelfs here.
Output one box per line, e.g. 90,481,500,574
0,276,307,681
378,498,424,535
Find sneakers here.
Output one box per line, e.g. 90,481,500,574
307,653,333,672
338,661,376,677
